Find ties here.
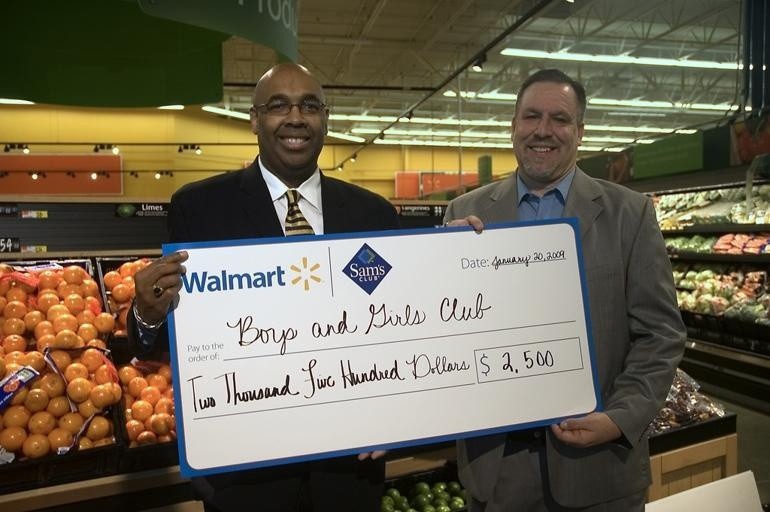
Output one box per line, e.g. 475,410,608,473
285,189,315,237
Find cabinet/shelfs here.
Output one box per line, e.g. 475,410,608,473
643,178,770,413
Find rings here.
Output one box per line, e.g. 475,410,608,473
444,221,450,228
152,281,164,298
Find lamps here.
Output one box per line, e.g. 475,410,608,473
472,56,483,73
338,165,344,172
350,155,359,164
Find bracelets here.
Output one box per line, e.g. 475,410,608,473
133,302,163,332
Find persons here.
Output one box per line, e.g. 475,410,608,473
441,69,688,511
126,63,394,512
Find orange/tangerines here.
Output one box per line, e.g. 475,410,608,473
384,482,465,512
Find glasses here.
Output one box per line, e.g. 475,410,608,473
251,97,328,115
